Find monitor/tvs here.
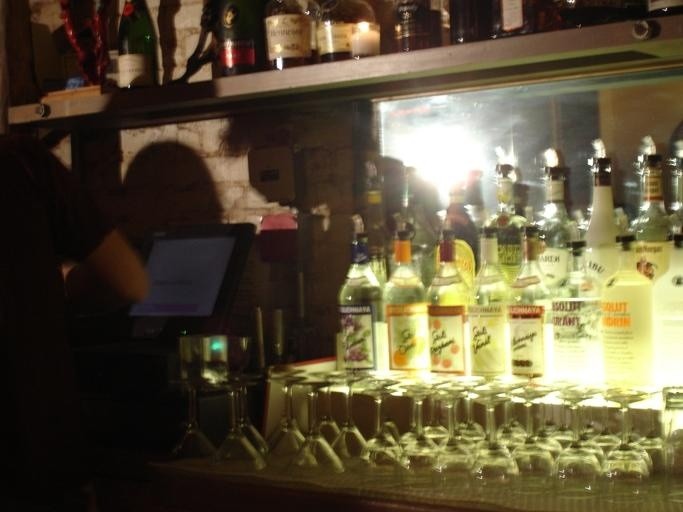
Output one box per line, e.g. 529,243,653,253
123,222,256,326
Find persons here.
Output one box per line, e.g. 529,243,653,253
0,133,151,512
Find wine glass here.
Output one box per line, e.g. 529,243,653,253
164,376,683,504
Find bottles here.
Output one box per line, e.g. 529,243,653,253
386,230,429,374
211,0,259,76
670,158,683,240
565,1,587,28
580,157,618,281
264,0,312,69
430,0,444,46
449,0,482,43
601,232,653,390
495,0,534,36
648,0,681,18
338,232,384,372
490,164,524,284
395,166,435,288
632,153,671,282
119,0,158,89
650,232,682,389
396,0,430,50
539,167,577,287
470,228,511,376
315,0,355,62
429,238,470,375
439,185,480,276
554,241,603,383
365,174,391,282
509,226,549,376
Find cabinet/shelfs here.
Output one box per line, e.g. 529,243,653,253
0,15,683,123
0,343,682,512
